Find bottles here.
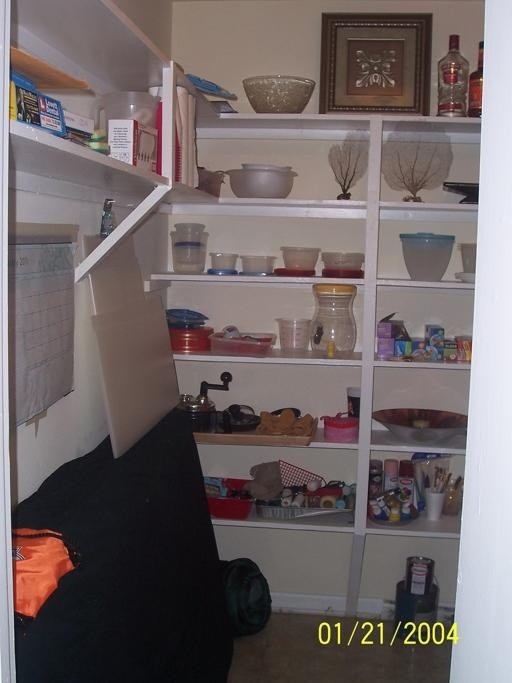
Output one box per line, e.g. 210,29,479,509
466,39,485,116
436,32,470,116
310,283,358,358
368,504,413,523
368,458,414,506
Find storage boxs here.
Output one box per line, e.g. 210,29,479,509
102,117,159,176
209,330,276,359
199,477,259,522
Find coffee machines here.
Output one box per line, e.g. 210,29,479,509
179,371,233,434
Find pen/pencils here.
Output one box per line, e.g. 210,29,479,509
434,468,452,493
377,488,401,501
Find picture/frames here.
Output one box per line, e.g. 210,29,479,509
317,8,433,118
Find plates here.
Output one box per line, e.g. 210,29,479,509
174,222,205,231
217,411,261,432
454,273,475,283
207,269,363,279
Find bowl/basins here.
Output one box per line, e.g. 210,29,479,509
209,242,365,273
370,407,469,441
241,75,318,112
222,159,299,197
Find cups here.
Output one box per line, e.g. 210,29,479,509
460,242,476,273
345,385,361,418
424,493,446,522
274,316,310,354
400,233,455,281
169,230,209,275
91,89,158,131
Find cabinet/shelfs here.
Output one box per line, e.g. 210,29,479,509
173,112,374,625
9,0,224,283
352,114,483,620
